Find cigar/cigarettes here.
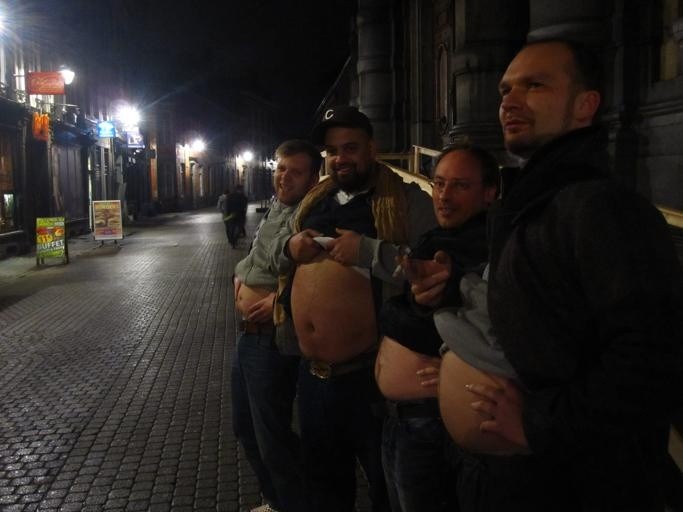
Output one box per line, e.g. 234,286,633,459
392,255,408,279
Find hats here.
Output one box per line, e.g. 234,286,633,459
311,105,371,136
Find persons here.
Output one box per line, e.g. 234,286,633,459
227,137,324,511
372,140,502,511
216,185,229,215
221,186,248,249
234,183,249,236
383,23,683,511
268,106,441,512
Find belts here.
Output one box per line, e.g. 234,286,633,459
383,397,441,418
304,343,373,381
239,320,274,333
